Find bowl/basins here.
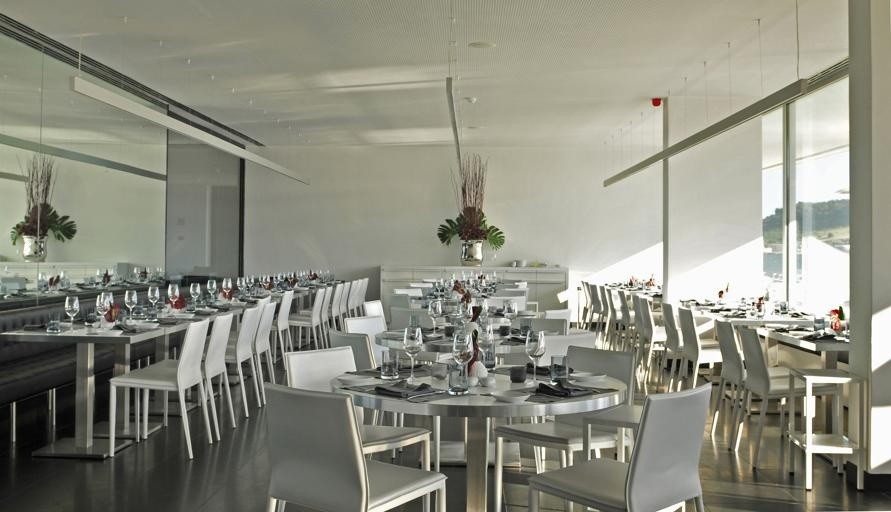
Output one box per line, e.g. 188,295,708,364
514,259,527,268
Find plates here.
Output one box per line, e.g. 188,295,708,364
571,371,606,383
338,374,375,385
492,390,530,403
96,329,123,336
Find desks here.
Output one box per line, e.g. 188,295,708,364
752,327,849,474
1,319,192,461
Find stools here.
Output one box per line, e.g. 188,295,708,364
788,367,865,494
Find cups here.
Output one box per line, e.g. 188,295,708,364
500,318,511,340
552,355,568,383
503,299,518,316
46,312,60,332
448,366,469,395
432,363,449,389
468,376,495,386
680,294,826,331
510,365,527,382
382,349,398,379
404,327,422,386
511,263,517,267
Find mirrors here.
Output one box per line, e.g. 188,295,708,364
0,33,168,312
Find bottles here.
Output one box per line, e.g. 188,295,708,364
39,269,97,295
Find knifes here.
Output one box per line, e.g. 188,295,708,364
406,389,445,400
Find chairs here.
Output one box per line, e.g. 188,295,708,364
108,277,805,512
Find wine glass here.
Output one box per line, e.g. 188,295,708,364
428,271,497,371
526,330,546,384
97,266,331,328
64,296,80,334
455,334,474,389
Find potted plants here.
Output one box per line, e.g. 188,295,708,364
10,151,77,264
437,151,506,267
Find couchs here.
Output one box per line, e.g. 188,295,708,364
0,287,192,444
